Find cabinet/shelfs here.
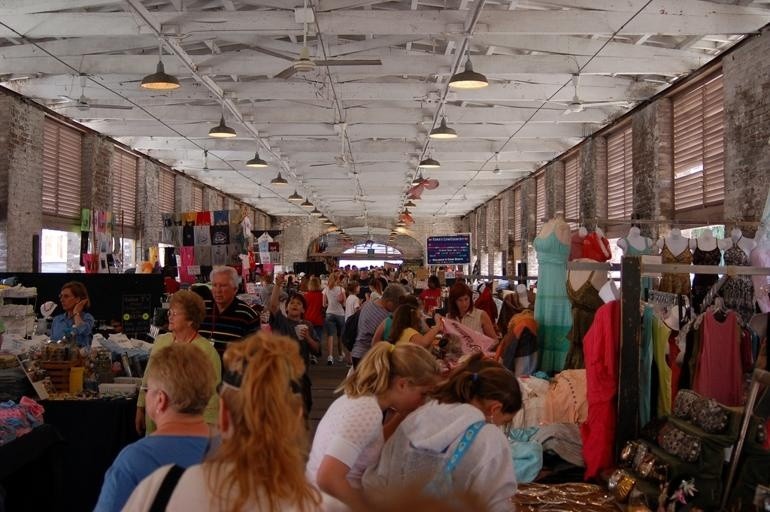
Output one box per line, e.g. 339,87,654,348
2,288,36,339
601,387,758,511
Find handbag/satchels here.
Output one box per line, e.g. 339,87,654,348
341,310,360,352
338,294,344,304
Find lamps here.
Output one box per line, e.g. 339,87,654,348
386,32,491,250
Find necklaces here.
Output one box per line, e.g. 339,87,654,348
43,304,54,312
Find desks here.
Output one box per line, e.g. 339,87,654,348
43,387,144,478
0,422,65,512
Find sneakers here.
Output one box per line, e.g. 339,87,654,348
346,362,352,368
328,356,334,366
337,352,345,362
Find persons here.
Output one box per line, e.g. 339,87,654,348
502,218,769,373
120,329,352,511
306,341,443,510
360,345,524,511
50,282,95,348
91,342,223,510
135,265,504,438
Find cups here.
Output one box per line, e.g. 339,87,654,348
295,325,307,339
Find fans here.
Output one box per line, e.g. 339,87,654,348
463,149,526,175
247,3,384,82
522,69,630,128
44,74,136,114
340,182,379,246
309,126,382,173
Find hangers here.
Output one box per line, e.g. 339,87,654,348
636,286,755,332
568,212,760,244
